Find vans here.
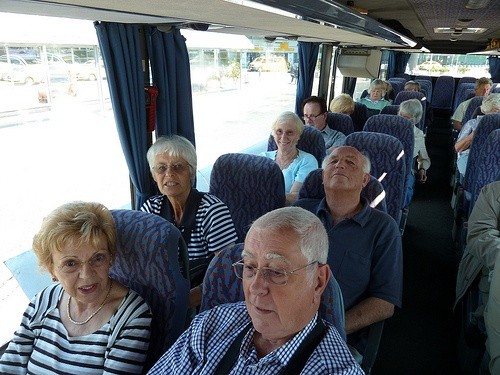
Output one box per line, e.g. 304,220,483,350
248,56,286,72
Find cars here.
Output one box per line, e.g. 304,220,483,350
418,60,443,70
0,51,107,84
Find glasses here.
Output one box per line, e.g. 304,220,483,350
152,162,189,173
232,259,320,288
51,249,111,273
301,113,322,121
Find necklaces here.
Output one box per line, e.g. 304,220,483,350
67,276,112,324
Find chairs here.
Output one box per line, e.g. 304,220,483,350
207,152,286,242
199,242,346,349
267,72,500,375
106,209,192,375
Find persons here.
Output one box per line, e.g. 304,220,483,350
455,93,500,184
145,206,367,375
256,110,319,207
289,144,404,366
450,77,494,138
330,93,364,132
356,79,396,115
302,96,347,156
0,200,152,375
403,80,420,92
452,180,500,375
137,135,238,320
398,99,431,215
